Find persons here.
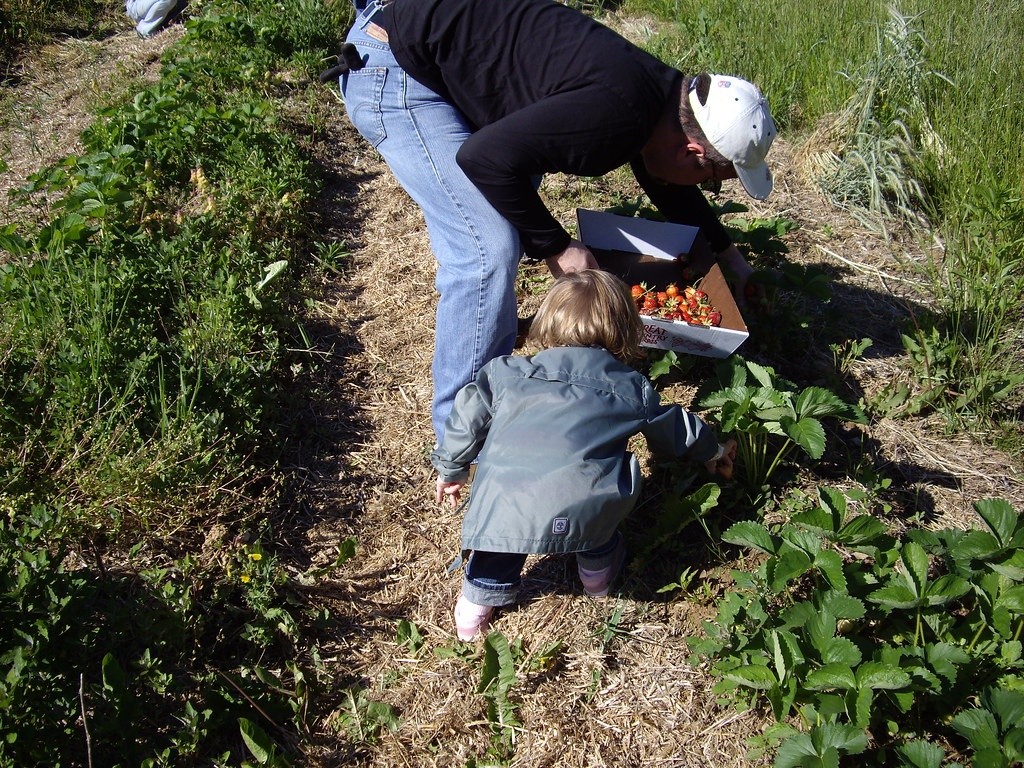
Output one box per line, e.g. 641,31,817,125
337,0,777,477
436,268,738,643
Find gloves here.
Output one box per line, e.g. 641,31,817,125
319,42,366,84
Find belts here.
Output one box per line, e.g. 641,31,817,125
366,22,389,43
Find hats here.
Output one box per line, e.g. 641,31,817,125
687,73,777,200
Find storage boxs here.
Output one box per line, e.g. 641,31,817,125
576,208,749,359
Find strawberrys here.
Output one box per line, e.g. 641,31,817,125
629,285,721,328
837,620,853,633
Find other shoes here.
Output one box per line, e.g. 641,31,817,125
576,561,620,598
452,594,496,643
